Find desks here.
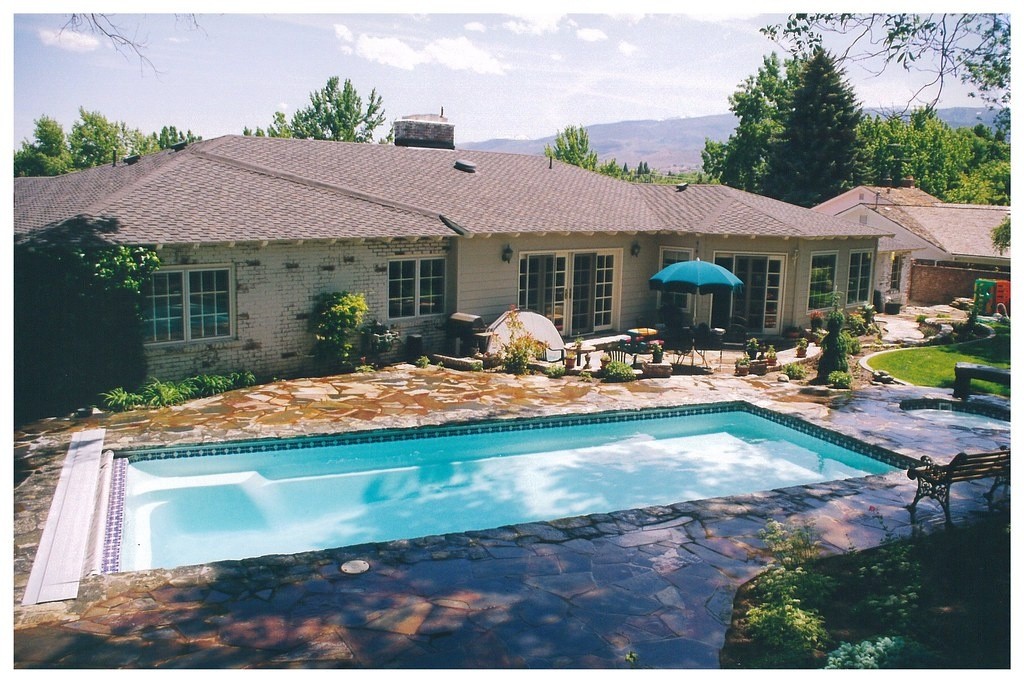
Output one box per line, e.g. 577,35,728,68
683,327,726,356
627,327,658,354
564,345,596,366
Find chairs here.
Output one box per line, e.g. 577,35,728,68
694,322,723,368
666,324,694,368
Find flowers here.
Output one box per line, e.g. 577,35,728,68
747,338,759,359
735,351,751,365
810,311,824,319
813,329,828,339
651,343,662,353
314,290,369,366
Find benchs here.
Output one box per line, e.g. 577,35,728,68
905,445,1011,525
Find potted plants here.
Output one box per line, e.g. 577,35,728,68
599,354,611,370
885,296,904,314
767,345,777,366
796,338,808,358
565,352,576,368
575,332,584,349
757,340,766,360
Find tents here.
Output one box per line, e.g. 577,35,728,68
485,309,567,363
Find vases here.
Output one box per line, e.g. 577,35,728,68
320,346,338,377
815,338,822,346
653,353,663,363
811,318,822,331
748,358,768,372
734,364,749,375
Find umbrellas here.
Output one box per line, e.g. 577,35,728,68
648,256,745,367
